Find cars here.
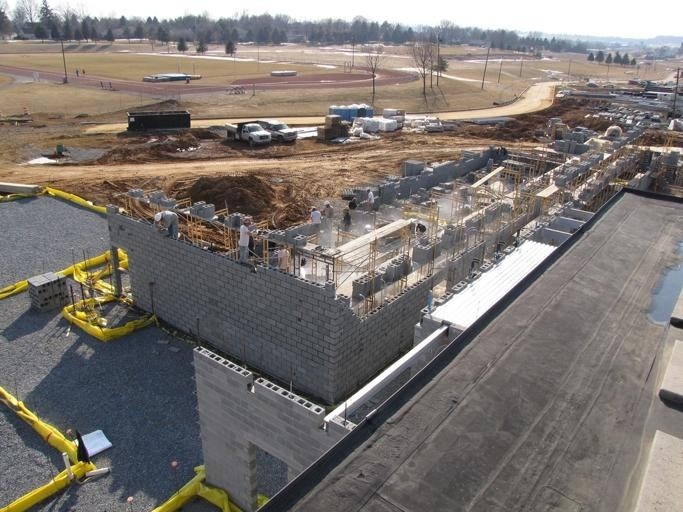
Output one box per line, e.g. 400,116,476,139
257,120,298,144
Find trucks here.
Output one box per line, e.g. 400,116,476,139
225,121,272,148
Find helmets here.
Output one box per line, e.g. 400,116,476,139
323,201,330,205
242,216,251,224
155,213,161,222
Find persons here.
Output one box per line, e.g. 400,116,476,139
324,201,333,235
155,211,178,238
277,245,289,271
311,206,322,237
238,218,257,264
367,189,374,212
344,197,357,231
416,223,426,232
